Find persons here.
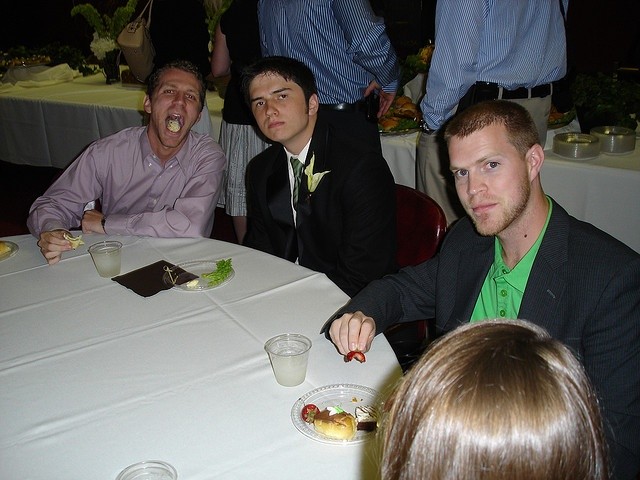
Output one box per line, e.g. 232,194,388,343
255,1,403,154
129,1,214,140
26,61,226,266
415,1,572,233
320,98,638,479
377,320,615,480
211,1,273,245
239,55,419,358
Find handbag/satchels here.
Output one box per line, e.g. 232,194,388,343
117,18,156,83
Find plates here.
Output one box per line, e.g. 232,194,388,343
545,112,575,130
378,123,421,138
0,240,19,263
290,384,386,448
6,55,53,68
591,124,637,156
552,132,601,161
162,259,232,292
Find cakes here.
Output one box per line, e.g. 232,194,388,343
355,405,378,431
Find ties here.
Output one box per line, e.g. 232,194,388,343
291,157,303,211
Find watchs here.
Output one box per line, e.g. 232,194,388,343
418,119,434,134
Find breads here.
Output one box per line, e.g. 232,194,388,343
315,410,357,440
384,121,398,130
379,117,392,125
396,96,412,108
401,104,417,113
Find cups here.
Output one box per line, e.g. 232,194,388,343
88,240,123,278
115,460,177,479
265,333,313,387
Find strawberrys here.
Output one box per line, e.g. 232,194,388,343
347,349,366,363
302,404,319,423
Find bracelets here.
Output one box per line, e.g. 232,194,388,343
101,215,109,236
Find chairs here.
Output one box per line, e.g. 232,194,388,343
394,182,447,341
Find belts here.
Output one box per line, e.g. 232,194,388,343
477,82,551,100
318,101,366,113
